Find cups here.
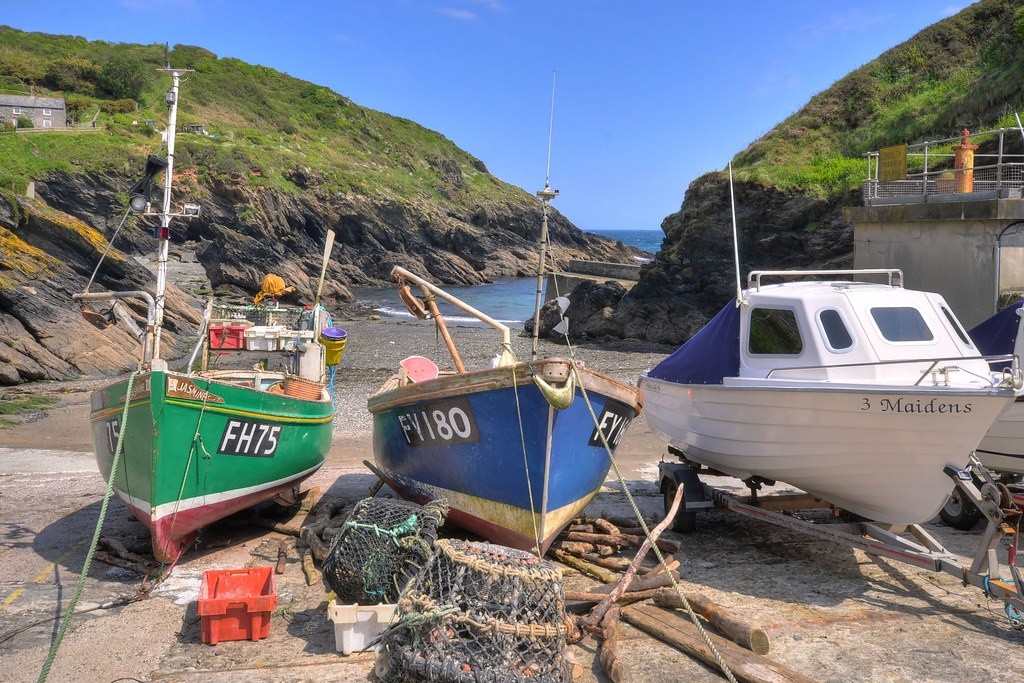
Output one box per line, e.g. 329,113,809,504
253,364,260,370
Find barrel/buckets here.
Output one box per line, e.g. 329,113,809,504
319,336,347,366
321,327,348,341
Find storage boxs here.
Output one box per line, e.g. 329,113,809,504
327,596,401,656
244,325,286,352
278,329,314,351
196,566,276,645
208,326,249,349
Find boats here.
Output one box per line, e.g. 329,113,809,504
87,66,351,561
369,68,646,558
635,160,1023,634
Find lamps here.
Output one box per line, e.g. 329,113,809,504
165,91,175,105
184,204,202,221
129,193,151,216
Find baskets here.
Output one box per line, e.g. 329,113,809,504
284,376,325,400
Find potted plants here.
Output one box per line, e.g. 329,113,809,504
935,168,956,192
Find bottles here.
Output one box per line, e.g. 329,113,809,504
297,333,302,344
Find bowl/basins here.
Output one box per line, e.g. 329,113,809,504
400,355,439,384
